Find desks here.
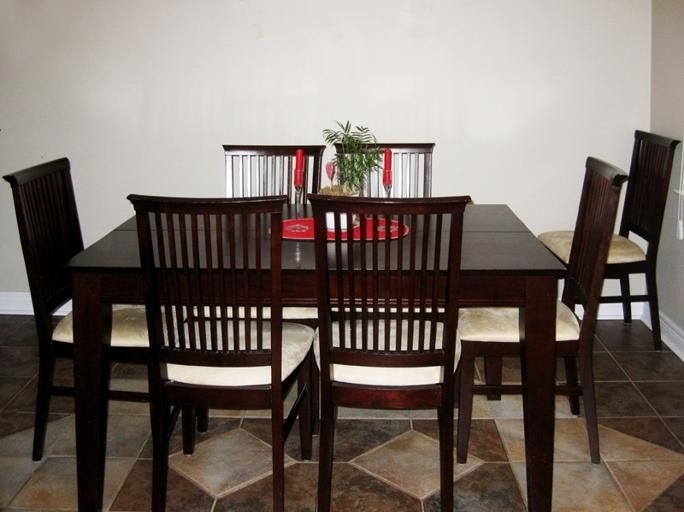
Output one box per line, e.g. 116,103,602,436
64,205,569,512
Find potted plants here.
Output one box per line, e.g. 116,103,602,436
322,118,386,224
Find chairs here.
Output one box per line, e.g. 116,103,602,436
190,144,326,438
333,142,445,318
2,156,188,461
126,191,315,511
538,129,681,351
456,155,629,464
305,192,472,511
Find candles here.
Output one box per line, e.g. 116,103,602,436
294,148,303,188
382,148,393,187
